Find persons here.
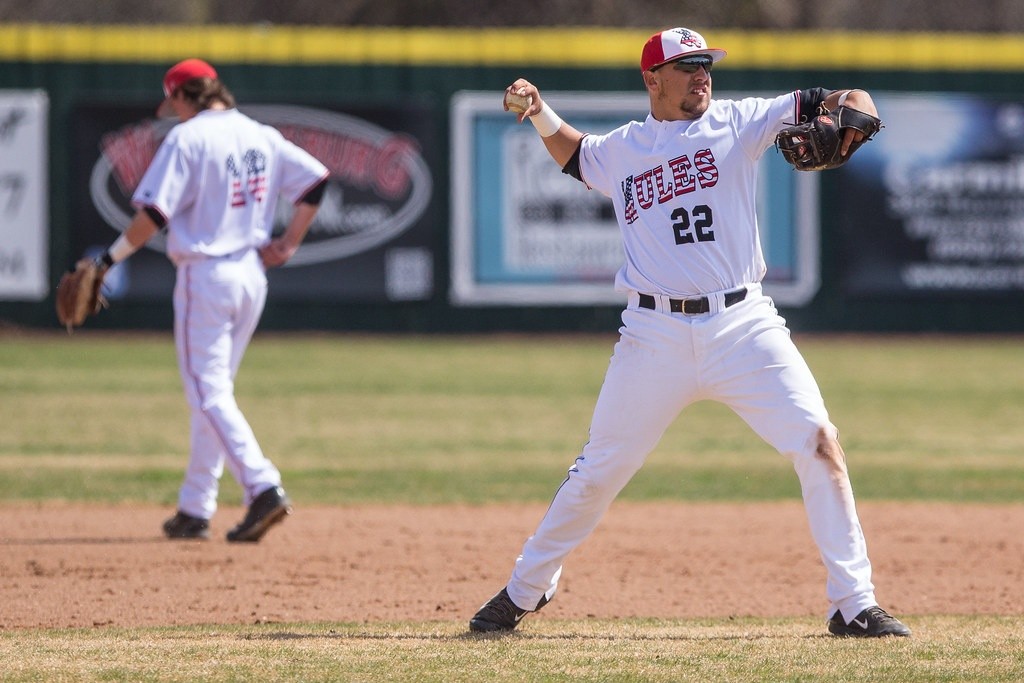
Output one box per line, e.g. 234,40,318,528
470,28,912,638
98,58,329,540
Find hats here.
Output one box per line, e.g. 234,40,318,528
157,58,217,118
641,28,727,73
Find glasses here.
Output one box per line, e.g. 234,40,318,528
673,54,714,74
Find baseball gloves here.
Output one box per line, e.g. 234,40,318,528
776,104,882,172
55,257,104,326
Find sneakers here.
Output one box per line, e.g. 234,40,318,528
163,511,209,539
470,584,558,632
829,607,912,636
227,487,291,542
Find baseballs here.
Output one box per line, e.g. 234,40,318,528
506,87,533,113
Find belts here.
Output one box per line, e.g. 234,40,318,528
639,289,748,316
